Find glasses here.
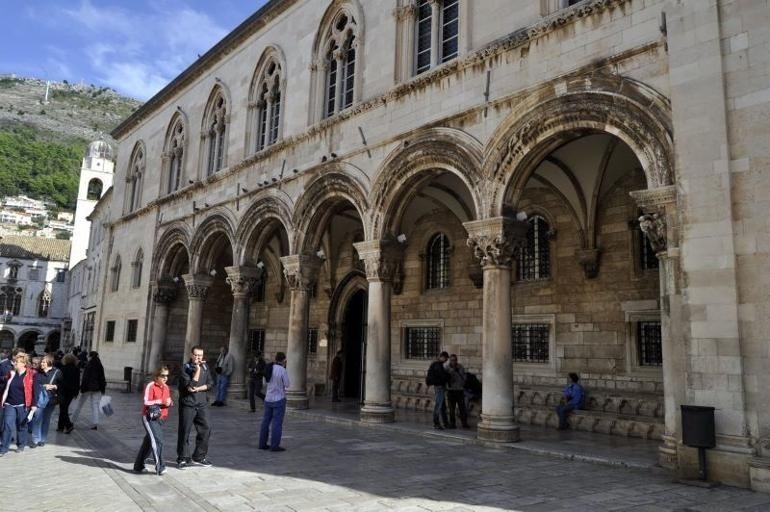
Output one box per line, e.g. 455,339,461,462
160,374,169,378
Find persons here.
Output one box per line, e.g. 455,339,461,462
0,342,108,459
258,352,291,454
178,344,218,470
211,343,236,409
132,365,177,478
422,350,481,433
554,371,585,433
327,348,345,404
243,351,268,414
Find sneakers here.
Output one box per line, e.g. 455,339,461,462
0,451,6,457
39,442,44,446
556,423,569,430
435,423,466,429
260,445,270,449
332,399,341,402
191,459,213,466
56,423,72,434
178,461,188,470
30,443,38,448
15,446,24,453
272,447,286,451
133,468,148,474
211,401,223,406
156,469,167,474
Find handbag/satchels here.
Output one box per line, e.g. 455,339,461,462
560,395,571,406
147,405,161,421
37,383,59,409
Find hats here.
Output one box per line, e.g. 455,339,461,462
89,351,99,357
37,391,49,409
